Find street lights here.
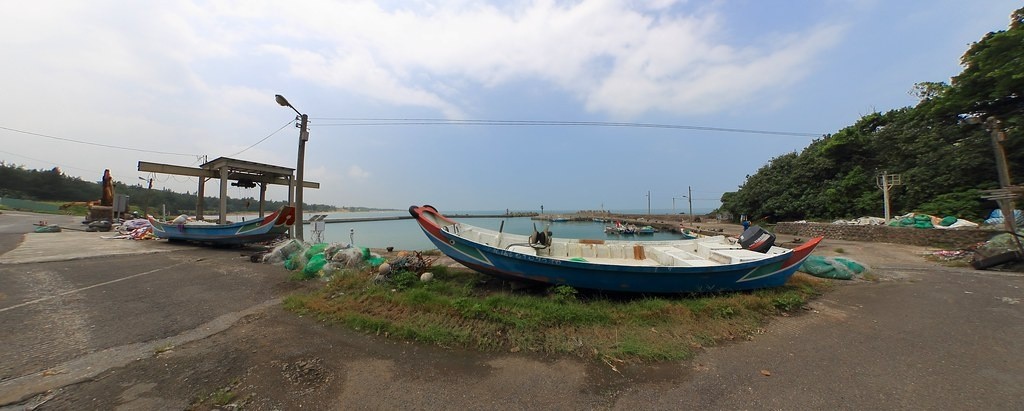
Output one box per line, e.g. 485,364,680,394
682,185,693,229
275,94,309,244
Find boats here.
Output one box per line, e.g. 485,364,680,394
146,204,297,242
408,204,826,297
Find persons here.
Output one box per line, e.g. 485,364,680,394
102,169,115,206
